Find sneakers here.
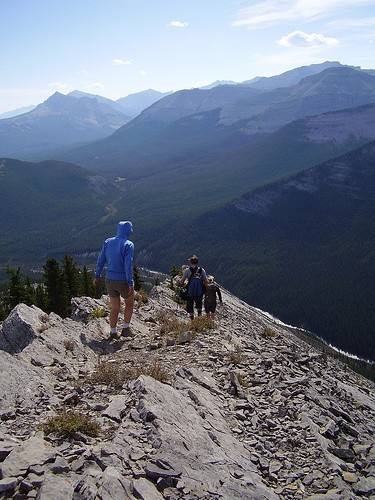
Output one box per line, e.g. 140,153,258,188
121,327,136,338
108,332,121,342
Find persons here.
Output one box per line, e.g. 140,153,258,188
92,221,137,340
176,254,208,321
201,275,223,323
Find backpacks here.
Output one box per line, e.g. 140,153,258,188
187,266,204,299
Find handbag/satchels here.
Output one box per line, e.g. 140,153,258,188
179,286,188,300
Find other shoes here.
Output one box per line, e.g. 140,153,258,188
190,315,194,322
198,310,202,317
